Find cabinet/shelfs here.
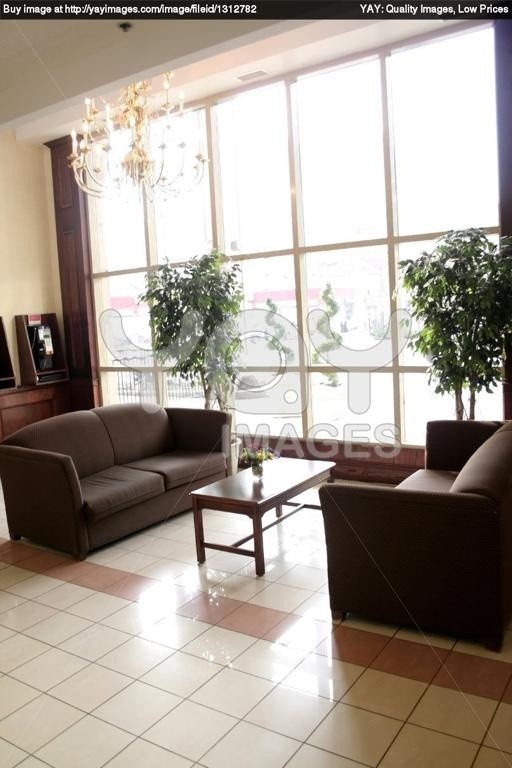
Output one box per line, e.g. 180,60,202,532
0,381,75,441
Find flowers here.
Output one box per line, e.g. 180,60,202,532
242,443,280,464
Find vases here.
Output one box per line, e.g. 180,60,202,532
251,459,264,477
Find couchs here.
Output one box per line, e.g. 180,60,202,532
0,402,231,561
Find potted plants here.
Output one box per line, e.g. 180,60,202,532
135,247,249,477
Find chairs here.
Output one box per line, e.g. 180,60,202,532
319,418,511,654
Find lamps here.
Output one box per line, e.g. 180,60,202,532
65,70,211,203
